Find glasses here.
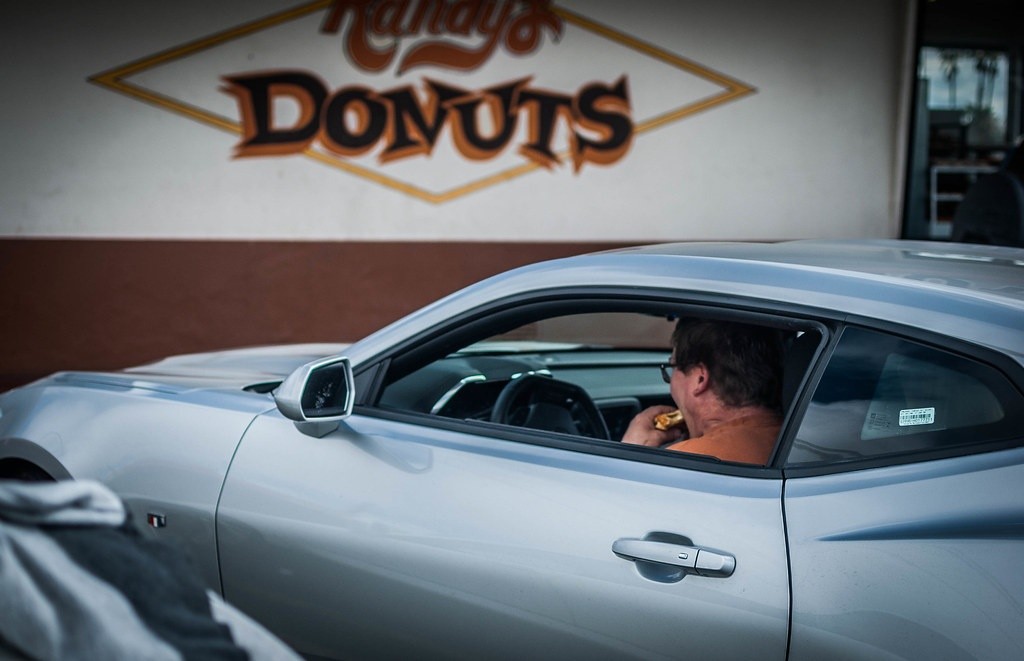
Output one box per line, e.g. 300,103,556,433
659,361,699,383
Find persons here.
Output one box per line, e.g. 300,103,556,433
619,315,787,466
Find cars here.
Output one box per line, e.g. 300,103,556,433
0,238,1024,661
